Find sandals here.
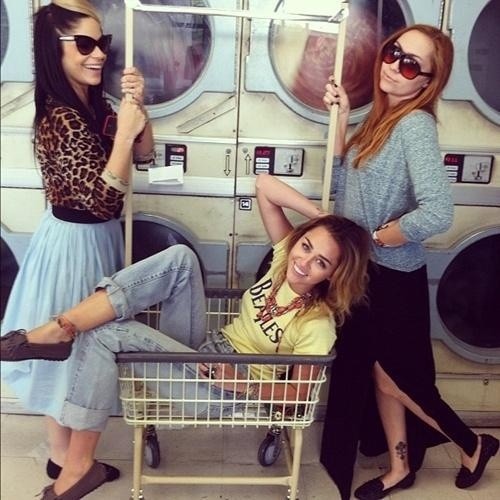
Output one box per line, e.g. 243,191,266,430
40,458,120,500
455,434,500,489
354,470,415,500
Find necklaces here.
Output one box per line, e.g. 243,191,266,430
256,277,315,322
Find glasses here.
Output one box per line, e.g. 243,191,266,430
383,44,432,82
61,35,112,54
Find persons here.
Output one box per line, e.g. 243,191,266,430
319,23,499,500
0,168,376,500
0,1,155,483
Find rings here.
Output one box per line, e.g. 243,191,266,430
207,368,216,376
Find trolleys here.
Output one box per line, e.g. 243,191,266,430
117,288,337,500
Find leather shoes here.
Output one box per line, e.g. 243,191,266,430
0,329,72,361
